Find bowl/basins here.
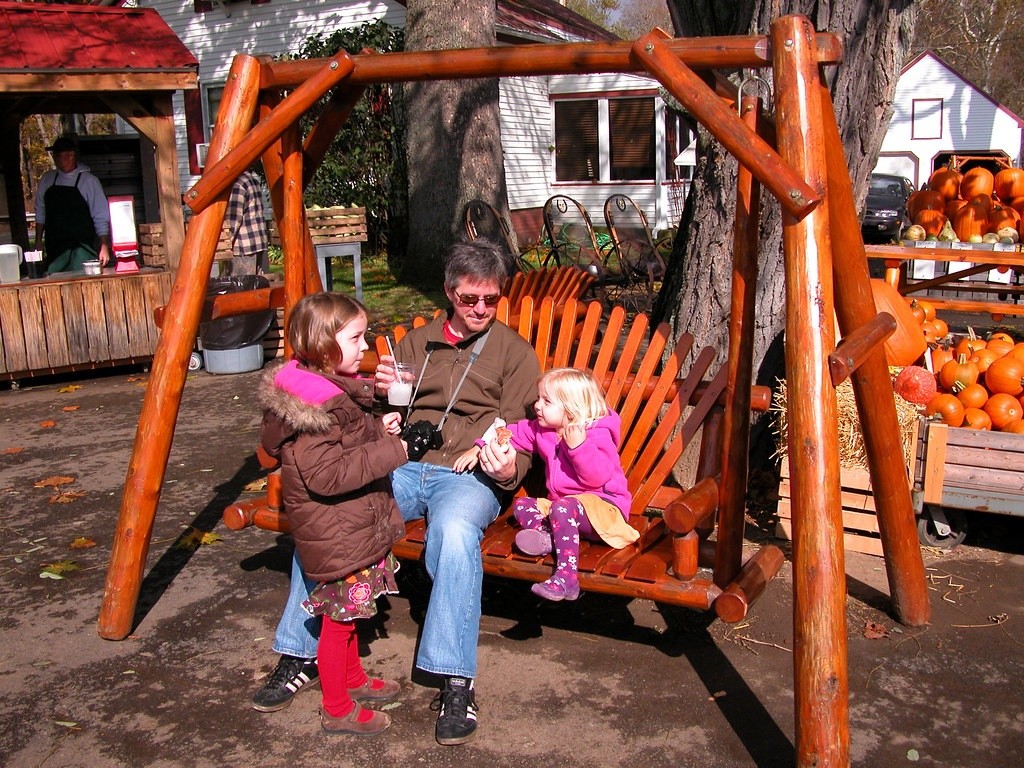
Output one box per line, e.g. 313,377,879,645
81,260,103,275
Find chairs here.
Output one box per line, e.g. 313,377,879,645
463,194,676,324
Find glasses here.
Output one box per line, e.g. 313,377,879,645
451,288,502,305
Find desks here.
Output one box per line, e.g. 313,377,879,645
315,242,364,302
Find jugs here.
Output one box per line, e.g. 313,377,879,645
0,244,23,282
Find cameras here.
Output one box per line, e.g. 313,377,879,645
401,419,444,462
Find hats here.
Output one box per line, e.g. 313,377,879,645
45,133,81,153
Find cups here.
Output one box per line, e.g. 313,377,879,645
384,361,416,406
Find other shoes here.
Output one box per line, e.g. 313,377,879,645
320,700,393,737
514,529,555,557
529,574,581,601
345,676,402,700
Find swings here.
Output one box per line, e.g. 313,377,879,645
223,65,785,623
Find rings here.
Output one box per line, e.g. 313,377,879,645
483,457,488,465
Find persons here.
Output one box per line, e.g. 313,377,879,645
477,367,641,601
35,138,111,275
250,236,542,745
254,293,441,736
225,170,274,278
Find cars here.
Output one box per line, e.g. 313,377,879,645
861,172,916,244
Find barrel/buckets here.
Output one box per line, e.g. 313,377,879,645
26,260,44,279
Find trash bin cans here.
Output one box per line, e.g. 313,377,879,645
201,274,266,375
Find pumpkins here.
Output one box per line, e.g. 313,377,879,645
867,276,1024,435
906,164,1024,244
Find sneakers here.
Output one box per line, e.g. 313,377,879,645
430,676,481,744
249,655,322,713
